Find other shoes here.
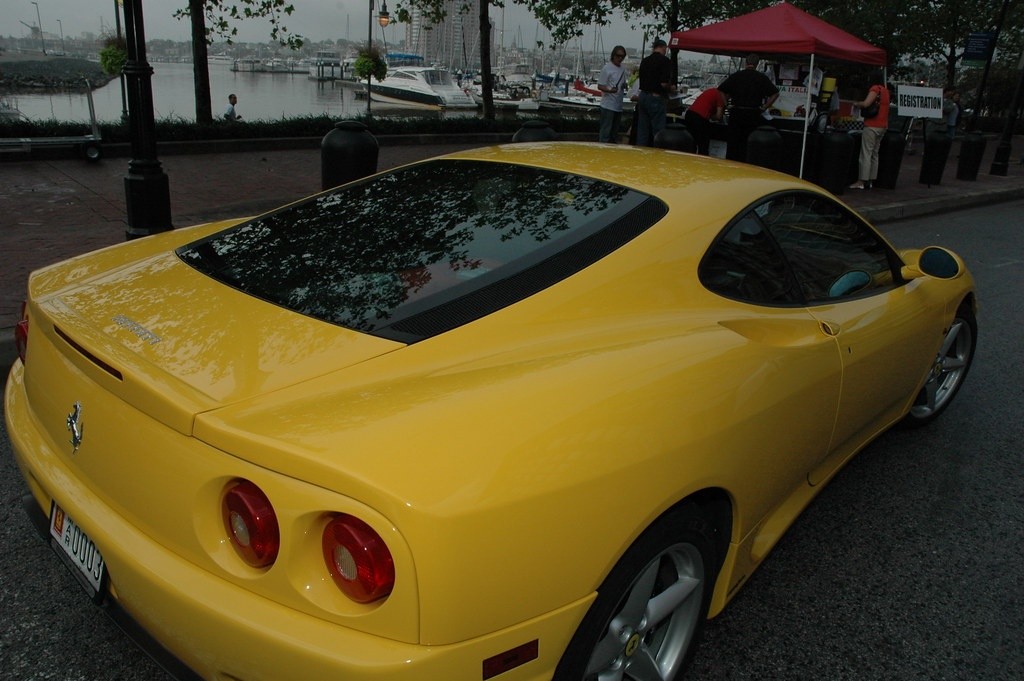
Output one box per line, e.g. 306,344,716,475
848,182,865,189
864,181,873,189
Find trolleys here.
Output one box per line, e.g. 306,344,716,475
0,75,105,161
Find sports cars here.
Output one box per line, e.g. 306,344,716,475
1,139,979,681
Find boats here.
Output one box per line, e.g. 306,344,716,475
0,56,121,94
0,100,21,118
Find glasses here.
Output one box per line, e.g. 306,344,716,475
615,54,624,58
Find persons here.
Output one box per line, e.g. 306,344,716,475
223,93,243,123
637,40,676,148
716,53,780,160
597,45,629,143
849,70,890,188
925,87,955,136
948,92,960,140
685,88,728,156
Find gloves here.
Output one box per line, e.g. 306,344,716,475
755,104,768,114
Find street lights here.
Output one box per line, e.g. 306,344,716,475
31,1,67,55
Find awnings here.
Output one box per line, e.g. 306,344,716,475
669,3,887,179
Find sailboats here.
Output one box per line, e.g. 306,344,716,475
206,1,747,119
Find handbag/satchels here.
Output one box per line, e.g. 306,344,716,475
861,83,881,117
